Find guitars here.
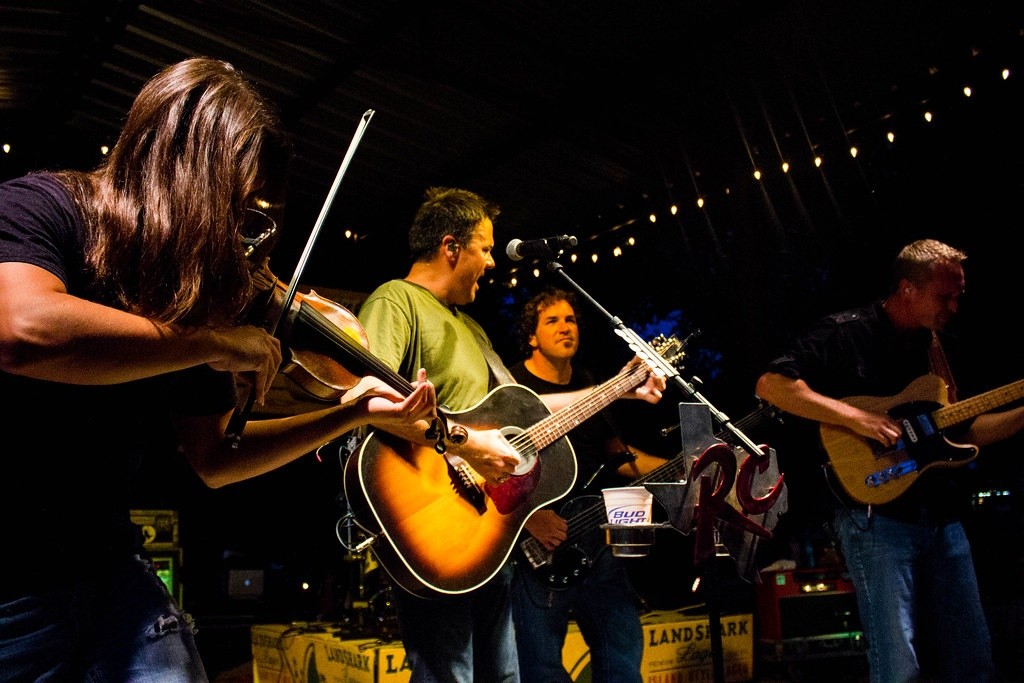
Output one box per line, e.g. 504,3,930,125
341,332,688,607
818,372,1023,511
512,401,786,596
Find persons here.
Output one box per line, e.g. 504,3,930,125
340,187,667,683
508,292,643,683
755,237,1024,683
0,55,434,683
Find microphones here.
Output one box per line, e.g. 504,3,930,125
507,234,577,261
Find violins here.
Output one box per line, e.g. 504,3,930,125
191,254,470,454
